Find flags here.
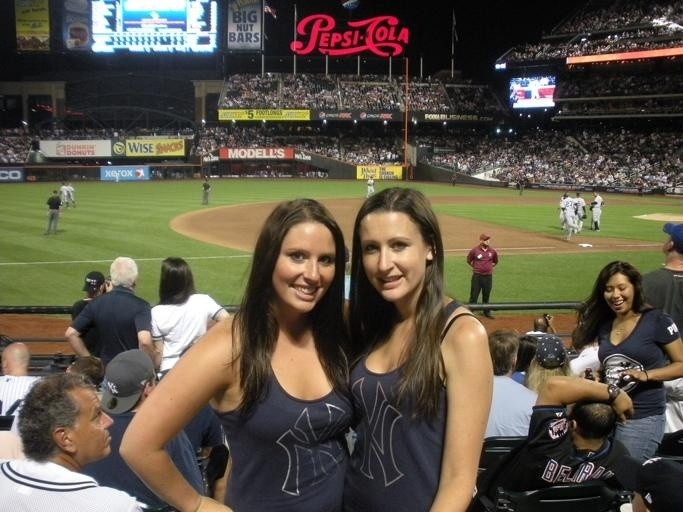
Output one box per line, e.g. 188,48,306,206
264,0,278,19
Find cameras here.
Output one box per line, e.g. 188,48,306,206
103,275,111,292
543,314,551,327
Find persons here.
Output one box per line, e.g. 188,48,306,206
58,182,70,208
558,196,605,243
464,234,501,319
42,190,63,236
1,67,494,181
493,1,683,197
65,182,77,205
366,175,375,199
466,260,683,512
337,186,496,512
639,222,682,336
118,196,357,512
451,171,458,187
2,255,230,511
201,179,212,206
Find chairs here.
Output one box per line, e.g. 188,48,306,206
474,437,619,512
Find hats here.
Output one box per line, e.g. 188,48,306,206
100,349,153,415
662,223,683,246
480,234,490,241
614,454,682,512
82,271,105,291
536,333,566,369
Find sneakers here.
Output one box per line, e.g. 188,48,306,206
486,316,496,319
